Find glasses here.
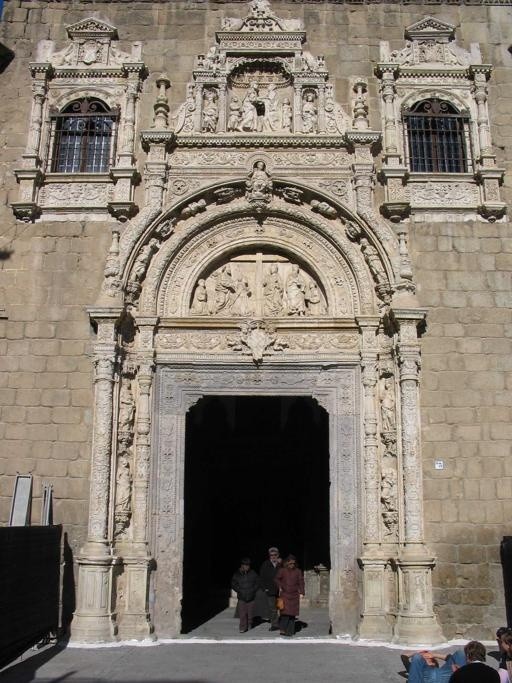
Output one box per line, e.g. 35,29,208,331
269,554,278,556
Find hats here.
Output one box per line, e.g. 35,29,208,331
268,547,279,554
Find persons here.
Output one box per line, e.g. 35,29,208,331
493,624,511,683
191,261,323,318
400,646,467,682
250,158,269,182
500,631,512,668
231,557,260,631
181,75,336,135
260,546,283,631
273,554,307,637
447,639,500,683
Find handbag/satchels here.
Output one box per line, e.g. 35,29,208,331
277,598,284,610
